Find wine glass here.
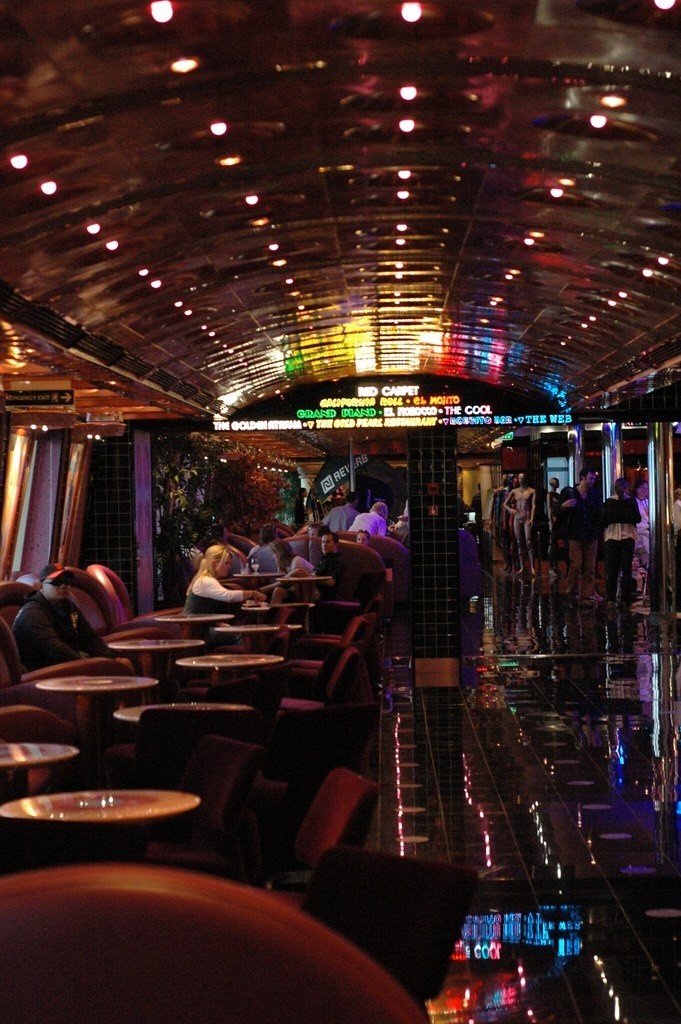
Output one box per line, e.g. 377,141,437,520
251,559,260,575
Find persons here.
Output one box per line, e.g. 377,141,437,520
489,473,562,578
179,488,388,650
559,467,681,614
12,564,136,752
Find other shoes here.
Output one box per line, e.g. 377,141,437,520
504,563,632,610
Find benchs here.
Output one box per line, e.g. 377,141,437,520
245,540,385,605
301,529,407,583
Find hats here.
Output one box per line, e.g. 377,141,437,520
39,564,74,581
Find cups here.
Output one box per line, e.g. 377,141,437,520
310,567,318,578
240,563,248,576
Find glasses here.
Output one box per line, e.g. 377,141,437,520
43,579,72,587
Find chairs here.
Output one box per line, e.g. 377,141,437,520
0,604,476,1024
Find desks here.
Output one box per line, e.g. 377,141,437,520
176,654,285,673
113,702,253,724
0,743,80,802
108,638,205,673
210,623,302,655
34,674,158,788
153,613,234,640
234,573,285,589
0,789,201,872
278,575,331,634
